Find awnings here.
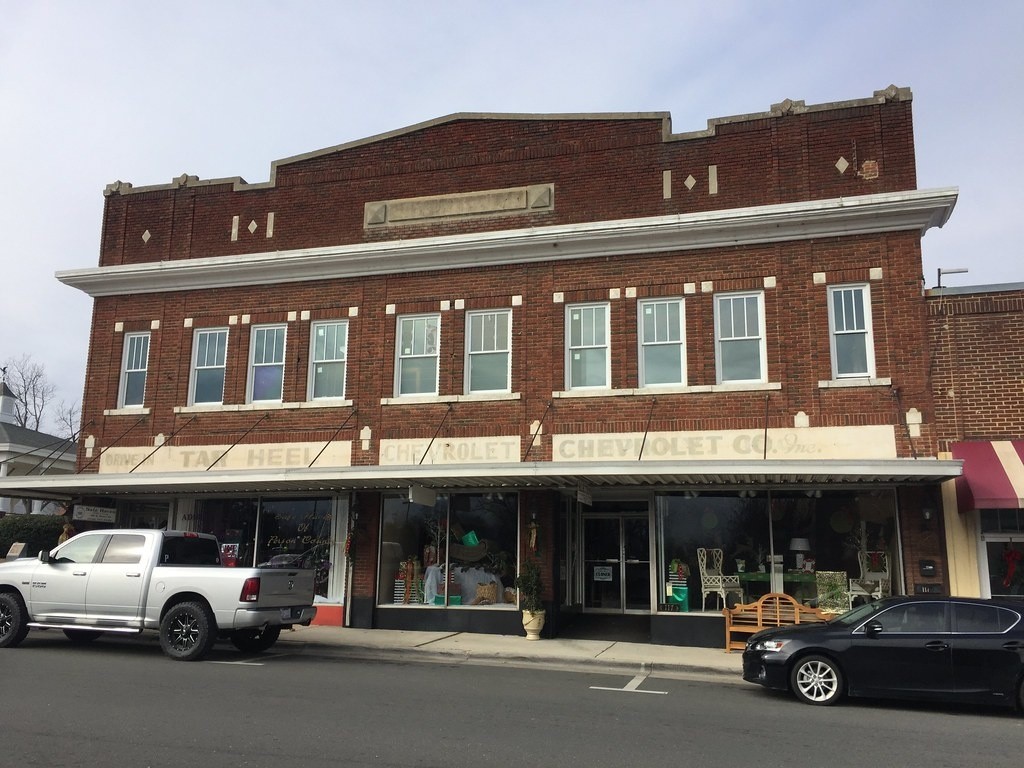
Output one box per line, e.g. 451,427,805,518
949,439,1024,513
1,458,966,505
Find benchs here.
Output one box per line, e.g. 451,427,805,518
722,593,836,654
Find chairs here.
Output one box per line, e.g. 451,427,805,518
848,550,892,611
697,548,745,612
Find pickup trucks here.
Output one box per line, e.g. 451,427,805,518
0,529,318,662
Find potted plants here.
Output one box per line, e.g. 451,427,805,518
514,556,548,641
735,559,745,572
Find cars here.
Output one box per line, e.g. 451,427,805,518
742,594,1024,718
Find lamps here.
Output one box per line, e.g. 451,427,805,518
789,538,810,569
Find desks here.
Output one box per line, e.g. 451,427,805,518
733,572,816,582
